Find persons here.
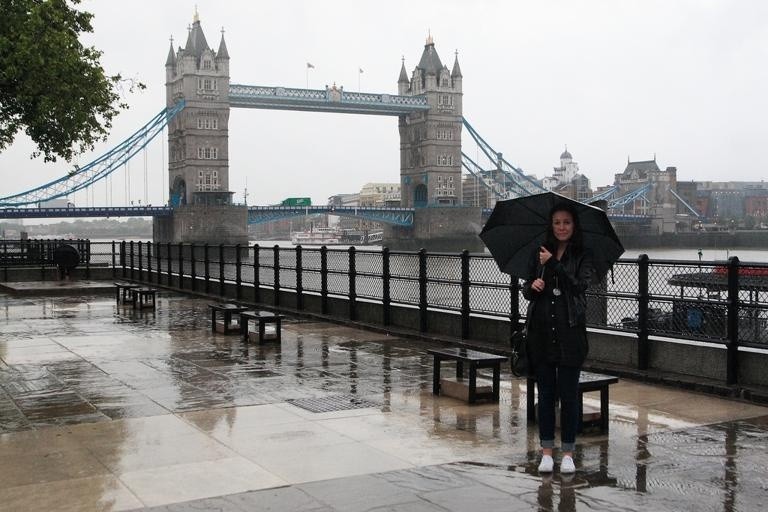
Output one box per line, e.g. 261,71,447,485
523,203,592,474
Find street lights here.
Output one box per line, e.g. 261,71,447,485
676,220,679,234
731,218,735,234
697,220,702,235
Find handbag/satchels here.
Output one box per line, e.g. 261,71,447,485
511,327,530,377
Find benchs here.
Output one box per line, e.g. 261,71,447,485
130,287,157,323
427,347,509,406
115,283,139,317
527,370,619,436
241,311,286,350
210,303,248,335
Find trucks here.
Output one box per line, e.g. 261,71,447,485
279,196,311,207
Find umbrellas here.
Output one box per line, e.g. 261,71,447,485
478,191,625,292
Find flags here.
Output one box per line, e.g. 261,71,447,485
308,63,314,69
360,69,363,73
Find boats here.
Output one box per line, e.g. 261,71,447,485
292,218,363,245
607,247,768,351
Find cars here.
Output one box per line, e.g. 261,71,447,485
753,222,768,230
691,224,706,231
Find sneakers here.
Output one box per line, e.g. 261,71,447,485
560,455,575,473
538,454,553,472
